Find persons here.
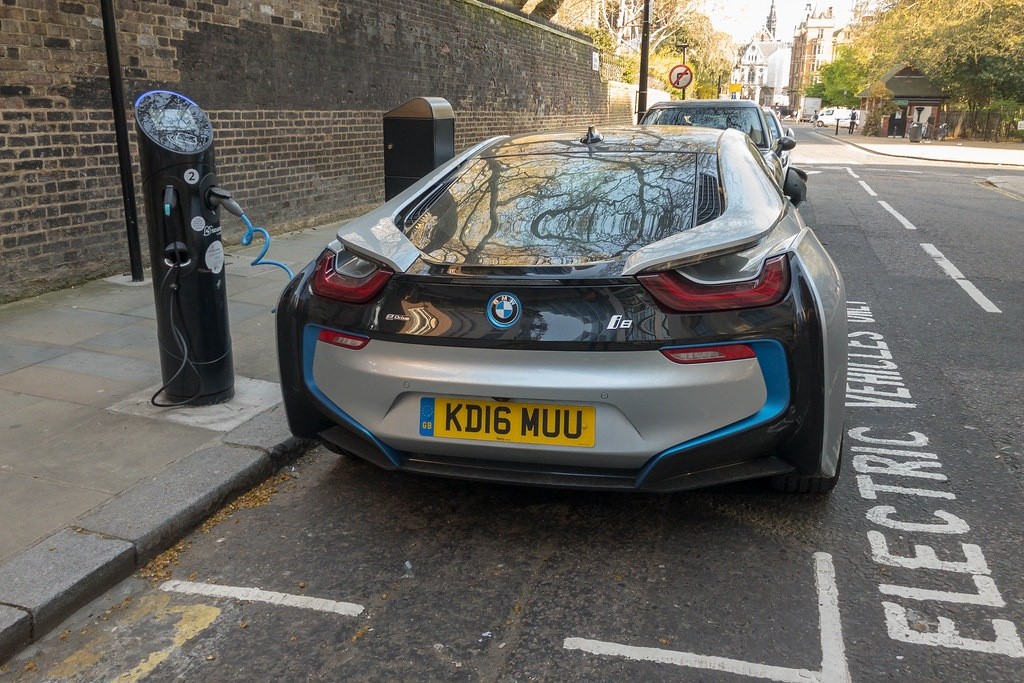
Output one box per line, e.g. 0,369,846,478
924,113,936,140
812,110,818,129
849,108,858,134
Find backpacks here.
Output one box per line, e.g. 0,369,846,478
851,111,856,120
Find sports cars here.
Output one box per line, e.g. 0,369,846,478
274,124,847,496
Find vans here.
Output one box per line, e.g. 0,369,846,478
817,108,860,129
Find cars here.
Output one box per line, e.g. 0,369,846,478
762,107,798,168
639,100,796,196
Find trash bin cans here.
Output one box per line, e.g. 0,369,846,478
909,123,922,143
383,96,456,203
